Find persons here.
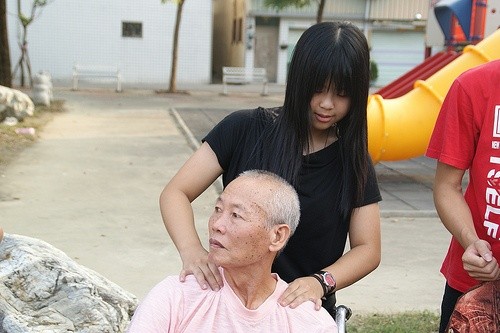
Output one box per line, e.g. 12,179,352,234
158,22,383,320
126,168,338,333
425,58,500,333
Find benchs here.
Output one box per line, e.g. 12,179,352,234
222,67,267,95
72,65,123,92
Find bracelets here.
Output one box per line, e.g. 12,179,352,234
312,275,326,297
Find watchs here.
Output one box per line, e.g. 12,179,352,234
315,271,336,294
